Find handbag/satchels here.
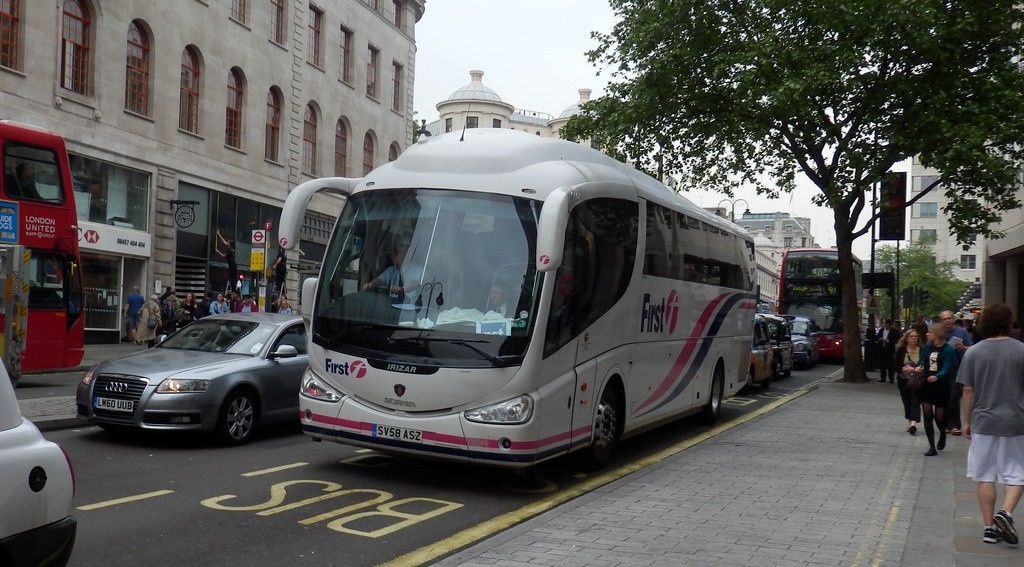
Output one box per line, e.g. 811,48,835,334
908,360,923,377
147,318,157,330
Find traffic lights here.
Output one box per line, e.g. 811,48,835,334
920,288,929,305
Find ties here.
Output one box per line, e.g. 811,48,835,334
497,307,502,313
397,271,405,303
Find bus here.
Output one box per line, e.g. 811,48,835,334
0,118,86,380
775,246,863,363
275,126,760,476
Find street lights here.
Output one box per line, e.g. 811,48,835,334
715,199,753,225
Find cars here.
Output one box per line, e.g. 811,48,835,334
76,312,308,445
740,315,772,392
763,312,794,378
0,358,78,566
788,317,815,367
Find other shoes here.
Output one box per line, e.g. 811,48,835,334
946,428,962,436
888,381,895,384
937,431,947,450
130,339,145,346
877,379,886,383
907,426,917,435
924,449,938,456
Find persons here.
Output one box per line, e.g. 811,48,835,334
363,245,428,302
482,280,528,320
956,303,1024,544
16,163,42,198
124,286,294,348
216,230,237,291
272,249,287,297
874,308,1024,456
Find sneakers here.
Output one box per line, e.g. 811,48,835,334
983,509,1019,545
